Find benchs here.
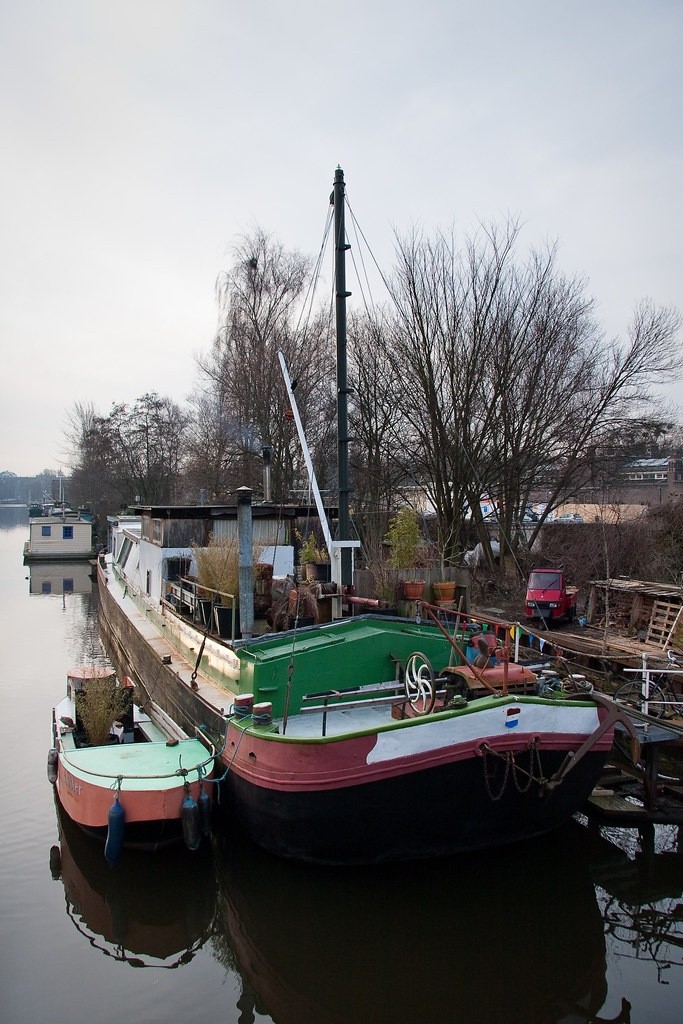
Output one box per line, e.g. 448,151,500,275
615,714,681,811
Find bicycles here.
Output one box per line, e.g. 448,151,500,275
613,650,683,729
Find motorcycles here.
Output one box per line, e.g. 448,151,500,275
525,568,579,630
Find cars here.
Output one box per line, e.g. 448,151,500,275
553,513,583,524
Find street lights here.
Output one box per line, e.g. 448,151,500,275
545,489,553,523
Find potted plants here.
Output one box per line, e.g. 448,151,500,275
189,532,282,640
265,572,321,632
72,667,141,748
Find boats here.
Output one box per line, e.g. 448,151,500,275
46,661,220,853
95,162,621,873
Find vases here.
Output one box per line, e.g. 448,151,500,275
404,580,425,599
432,581,456,600
